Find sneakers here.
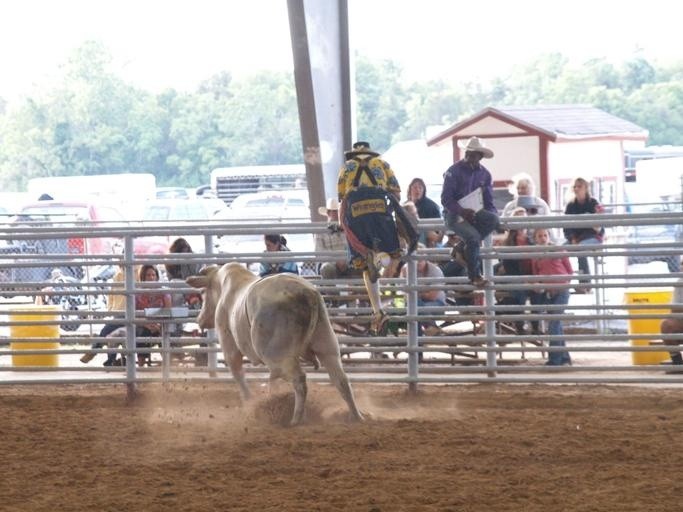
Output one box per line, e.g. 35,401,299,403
576,286,592,293
81,353,96,363
375,311,389,335
473,277,489,288
366,250,381,284
451,247,465,268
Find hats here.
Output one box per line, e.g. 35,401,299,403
318,198,340,216
343,142,381,161
456,135,494,159
508,195,544,213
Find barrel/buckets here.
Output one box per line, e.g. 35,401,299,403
9,304,60,370
624,287,674,365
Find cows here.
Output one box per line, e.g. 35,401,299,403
183,264,364,427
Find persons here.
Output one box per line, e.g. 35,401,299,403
661,233,682,374
79,237,208,366
530,228,571,366
561,179,605,291
259,232,298,273
336,142,402,337
439,137,509,289
320,174,550,335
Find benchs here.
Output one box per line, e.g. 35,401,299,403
116,201,615,378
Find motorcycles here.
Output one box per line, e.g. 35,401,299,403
38,264,116,332
